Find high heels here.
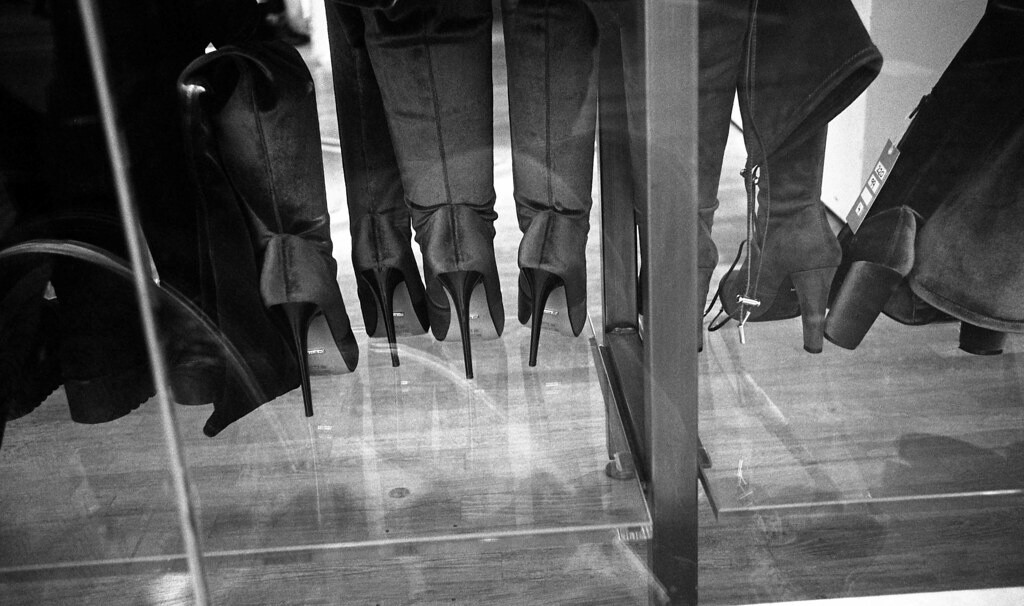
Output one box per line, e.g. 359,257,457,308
819,208,916,352
885,268,1007,355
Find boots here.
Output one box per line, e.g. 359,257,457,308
325,1,429,367
2,1,159,428
362,0,504,380
498,0,600,366
615,0,745,353
703,0,882,353
177,0,357,417
